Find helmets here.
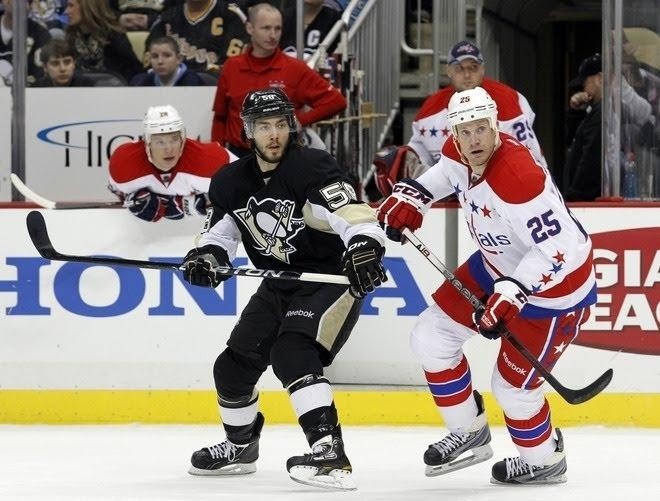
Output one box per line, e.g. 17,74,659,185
240,87,302,140
448,88,498,139
142,104,185,147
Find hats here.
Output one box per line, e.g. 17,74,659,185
565,52,602,87
447,41,484,67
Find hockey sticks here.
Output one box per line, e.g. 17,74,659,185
399,224,615,405
27,211,355,287
9,170,132,209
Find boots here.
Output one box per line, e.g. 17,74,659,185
424,389,492,466
190,412,264,470
492,428,567,484
286,423,352,477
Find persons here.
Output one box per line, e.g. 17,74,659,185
105,104,241,222
376,84,597,483
0,1,660,198
179,86,388,477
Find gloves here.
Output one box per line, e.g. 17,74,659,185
375,177,434,244
341,234,389,300
127,189,207,223
177,243,234,289
471,277,530,339
